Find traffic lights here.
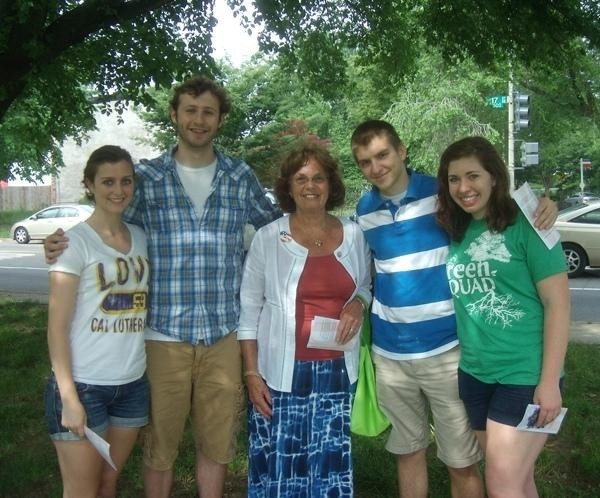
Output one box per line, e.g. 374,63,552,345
517,93,531,128
521,141,540,168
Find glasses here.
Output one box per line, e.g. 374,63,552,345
294,175,331,185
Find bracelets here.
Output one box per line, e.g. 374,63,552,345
243,370,260,376
356,298,365,311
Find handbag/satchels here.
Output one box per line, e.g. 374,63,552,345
350,309,394,438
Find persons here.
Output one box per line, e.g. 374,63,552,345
433,137,571,498
45,78,283,498
236,145,373,498
44,145,151,498
347,120,557,498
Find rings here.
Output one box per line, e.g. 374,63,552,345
68,430,72,432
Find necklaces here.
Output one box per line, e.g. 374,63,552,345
315,239,324,248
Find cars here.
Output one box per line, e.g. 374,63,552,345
11,204,95,244
553,192,600,279
